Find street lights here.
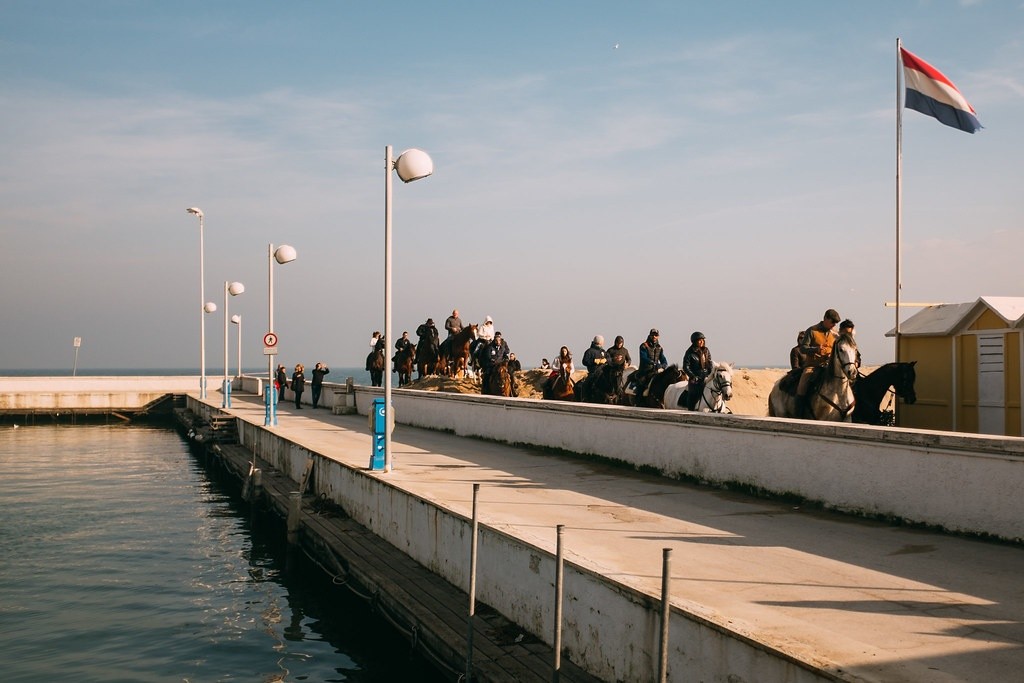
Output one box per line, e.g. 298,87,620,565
269,243,297,429
383,144,434,474
230,315,242,379
224,280,245,409
186,207,217,399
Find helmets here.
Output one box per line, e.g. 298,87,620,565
690,331,706,342
615,335,625,346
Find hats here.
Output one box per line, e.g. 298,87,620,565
424,318,435,325
650,328,659,334
825,308,842,324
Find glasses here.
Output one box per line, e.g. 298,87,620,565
651,334,659,337
799,336,804,341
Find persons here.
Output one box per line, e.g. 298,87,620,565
274,310,520,409
541,328,713,414
790,309,861,420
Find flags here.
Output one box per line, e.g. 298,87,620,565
901,46,982,134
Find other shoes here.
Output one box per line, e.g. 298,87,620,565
296,405,303,409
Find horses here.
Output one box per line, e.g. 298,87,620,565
480,359,689,409
366,323,480,388
768,330,919,427
663,360,734,414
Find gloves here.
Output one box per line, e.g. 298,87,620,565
594,358,607,365
